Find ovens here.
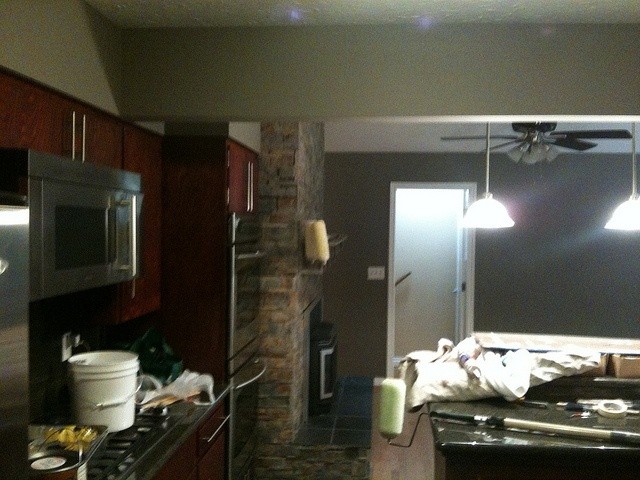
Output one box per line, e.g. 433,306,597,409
224,209,269,361
229,345,268,477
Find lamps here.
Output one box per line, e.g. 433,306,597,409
461,190,516,230
603,190,640,234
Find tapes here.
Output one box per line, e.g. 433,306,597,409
598,400,628,419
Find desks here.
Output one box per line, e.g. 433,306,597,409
425,400,639,479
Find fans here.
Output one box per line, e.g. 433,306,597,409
439,122,633,164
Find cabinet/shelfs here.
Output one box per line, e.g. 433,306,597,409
91,118,161,325
151,396,229,480
1,66,125,169
163,134,263,213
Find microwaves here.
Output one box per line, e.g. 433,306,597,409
0,146,147,304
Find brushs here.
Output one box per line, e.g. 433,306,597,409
380,378,640,447
